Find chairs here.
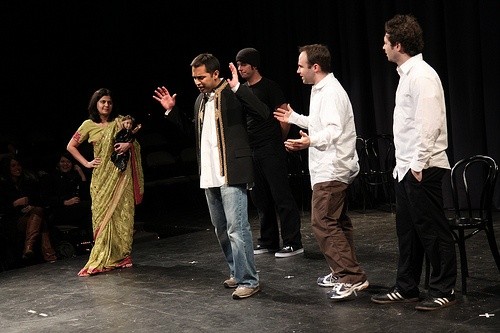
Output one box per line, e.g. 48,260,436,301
346,136,394,216
425,154,500,295
288,152,312,215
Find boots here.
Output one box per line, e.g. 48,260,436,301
22,214,42,259
40,232,58,264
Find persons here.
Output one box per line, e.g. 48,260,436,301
66,88,144,276
153,54,270,299
0,152,92,263
235,48,304,257
111,115,141,170
273,44,369,298
371,16,456,310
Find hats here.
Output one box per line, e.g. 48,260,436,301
236,47,261,69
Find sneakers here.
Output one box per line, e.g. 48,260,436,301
416,292,456,311
327,279,370,299
224,275,237,288
317,272,337,287
274,243,305,257
231,285,259,299
370,285,420,304
252,243,282,255
